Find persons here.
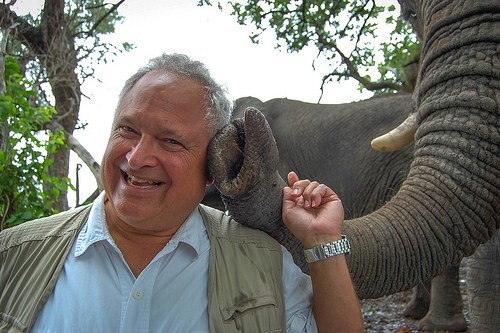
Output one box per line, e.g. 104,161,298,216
0,53,365,333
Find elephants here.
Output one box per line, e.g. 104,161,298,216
200,2,500,333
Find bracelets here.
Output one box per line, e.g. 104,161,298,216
302,233,351,263
